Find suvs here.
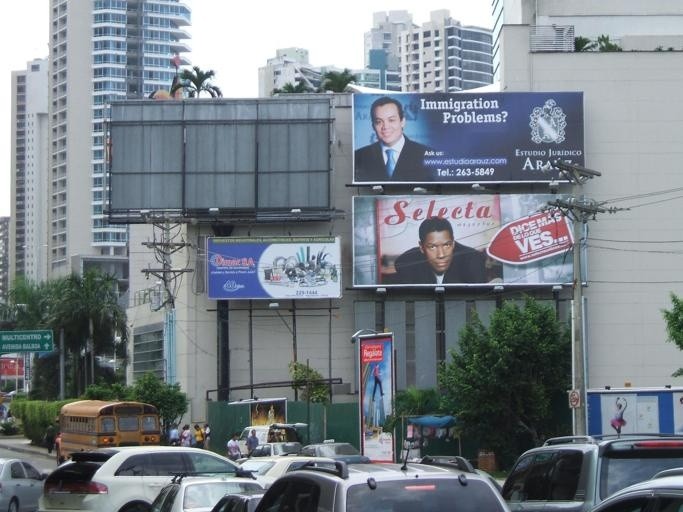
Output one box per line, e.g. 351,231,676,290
35,443,278,512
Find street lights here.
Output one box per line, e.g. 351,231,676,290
21,243,49,286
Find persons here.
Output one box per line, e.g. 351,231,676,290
44,424,54,453
182,425,190,446
193,424,203,449
203,423,211,450
244,430,258,453
395,217,484,284
371,365,384,402
7,409,12,423
267,404,275,423
607,397,627,439
353,97,435,182
168,423,180,446
225,433,241,460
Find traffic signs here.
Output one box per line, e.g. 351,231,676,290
0,330,53,353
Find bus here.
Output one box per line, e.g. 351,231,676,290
51,397,163,468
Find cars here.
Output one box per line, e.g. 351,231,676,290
0,387,25,437
0,455,48,512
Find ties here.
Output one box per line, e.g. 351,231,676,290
385,148,397,178
